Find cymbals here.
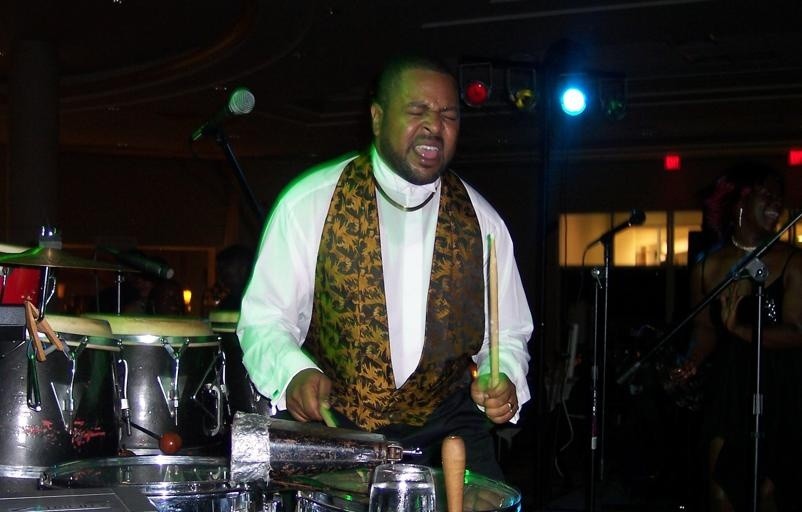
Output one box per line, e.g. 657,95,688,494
0,247,140,272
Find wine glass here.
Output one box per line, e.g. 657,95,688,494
369,463,437,512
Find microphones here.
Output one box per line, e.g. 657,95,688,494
99,241,174,280
187,88,256,145
599,206,647,242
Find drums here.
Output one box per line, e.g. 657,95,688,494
1,314,123,491
208,309,277,414
38,456,284,512
79,313,227,457
295,463,522,512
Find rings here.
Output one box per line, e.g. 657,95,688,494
506,401,513,410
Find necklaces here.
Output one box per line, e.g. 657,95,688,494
372,171,441,214
728,235,758,252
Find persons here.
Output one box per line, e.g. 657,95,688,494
86,243,250,315
235,56,535,484
665,165,802,510
